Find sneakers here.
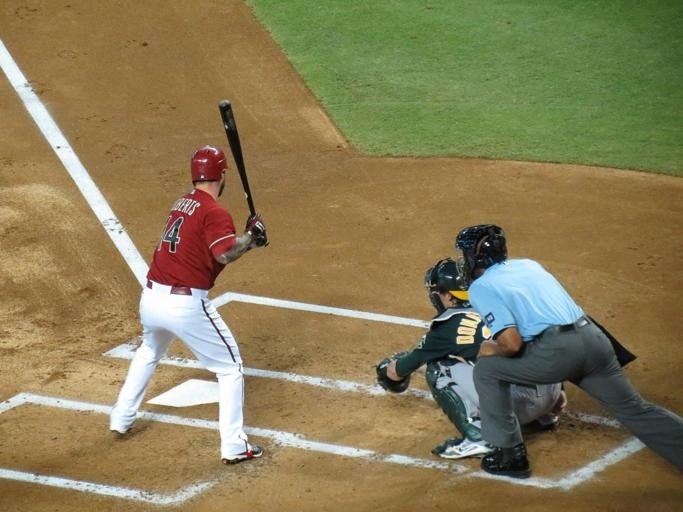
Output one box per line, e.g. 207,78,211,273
439,441,532,483
220,445,264,465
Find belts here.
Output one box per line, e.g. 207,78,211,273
539,317,593,336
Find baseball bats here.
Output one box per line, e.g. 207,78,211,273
217,100,255,220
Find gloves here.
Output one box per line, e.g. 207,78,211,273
245,214,270,250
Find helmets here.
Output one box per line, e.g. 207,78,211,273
424,257,469,312
190,146,228,181
456,223,510,292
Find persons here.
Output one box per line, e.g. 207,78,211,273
107,144,266,465
371,255,568,461
451,221,681,479
